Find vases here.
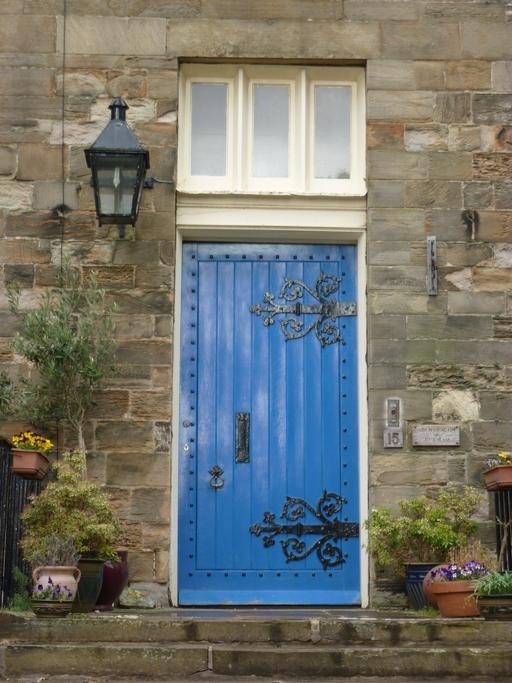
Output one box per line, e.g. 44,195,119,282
95,546,129,610
483,466,512,491
12,449,50,481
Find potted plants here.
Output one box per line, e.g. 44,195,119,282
18,448,127,619
360,481,511,621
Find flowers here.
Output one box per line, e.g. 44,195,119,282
482,449,512,472
12,430,54,454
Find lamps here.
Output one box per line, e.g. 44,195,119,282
84,97,154,240
